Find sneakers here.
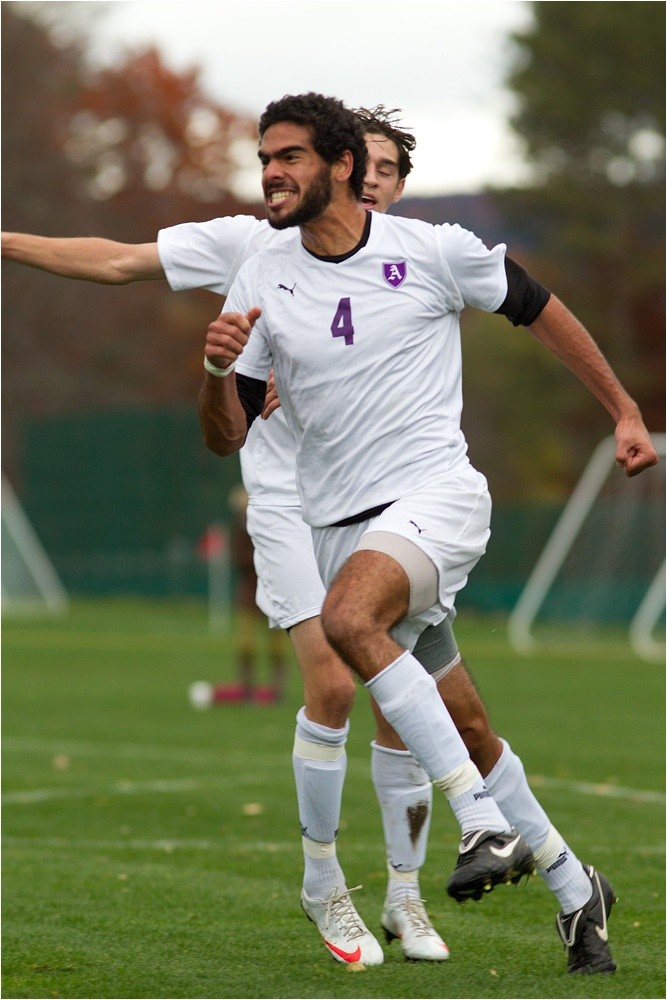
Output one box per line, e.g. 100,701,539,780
381,878,455,963
446,826,535,906
300,884,384,969
557,863,622,977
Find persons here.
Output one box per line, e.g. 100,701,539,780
199,92,659,976
211,490,287,703
1,103,453,965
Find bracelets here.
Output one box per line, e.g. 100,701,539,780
204,354,237,376
